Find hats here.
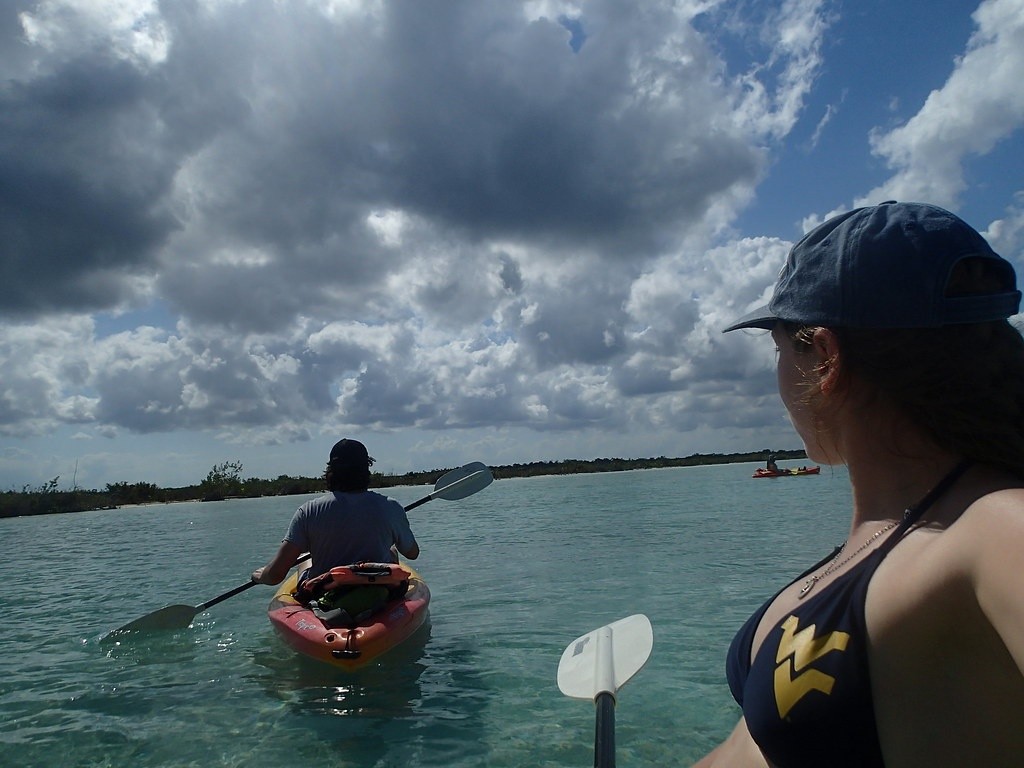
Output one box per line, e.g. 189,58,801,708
330,438,368,467
723,200,1020,333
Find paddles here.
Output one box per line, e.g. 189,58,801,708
557,613,655,768
101,460,494,641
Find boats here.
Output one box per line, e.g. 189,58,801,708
264,558,432,674
752,465,821,478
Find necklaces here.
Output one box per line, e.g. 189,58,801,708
798,478,933,598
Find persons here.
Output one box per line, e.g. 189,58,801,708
250,437,419,606
694,200,1023,766
765,455,807,474
